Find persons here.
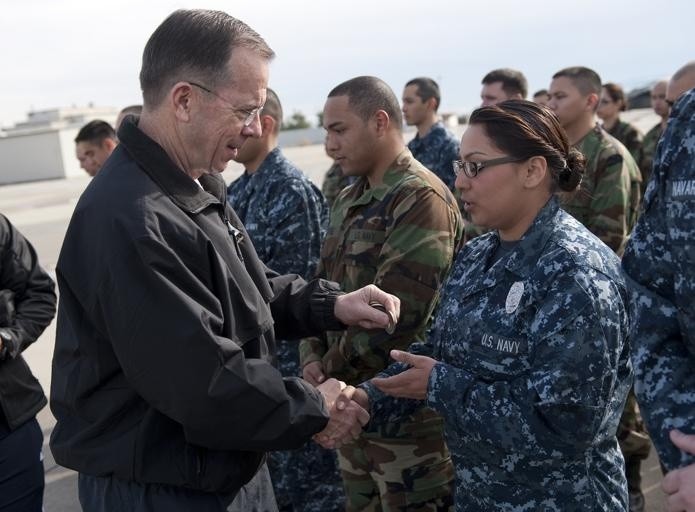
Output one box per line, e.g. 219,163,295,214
74,64,694,510
0,214,57,511
50,11,401,511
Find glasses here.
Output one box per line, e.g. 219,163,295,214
452,157,520,178
190,82,264,128
